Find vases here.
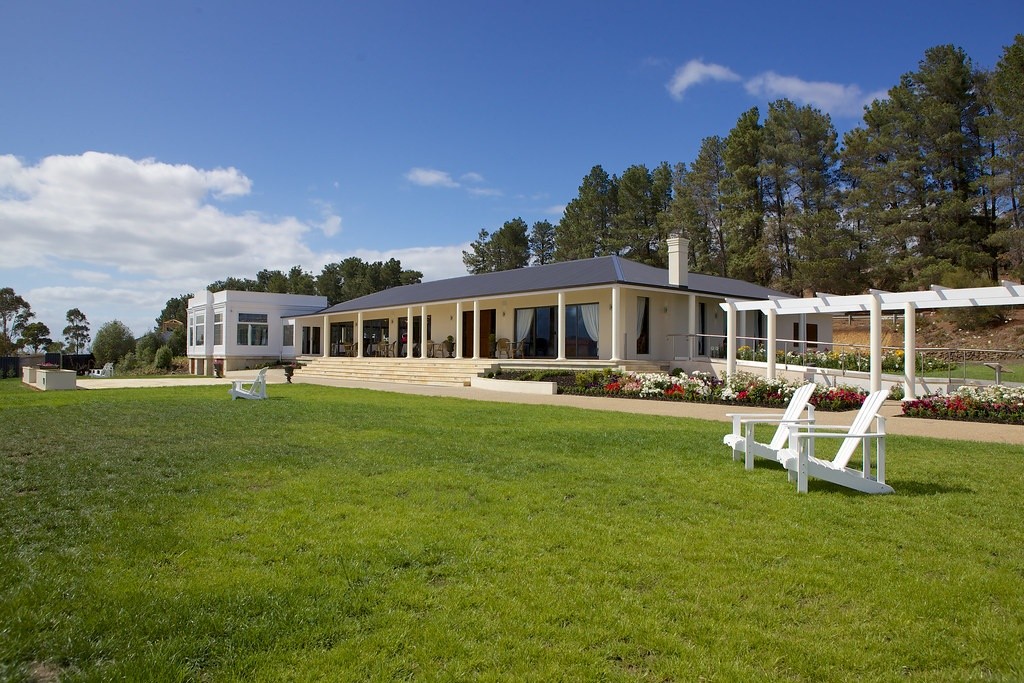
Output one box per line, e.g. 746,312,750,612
36,365,60,369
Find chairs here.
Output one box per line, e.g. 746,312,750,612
776,389,895,494
88,362,114,377
228,366,269,401
723,382,816,470
